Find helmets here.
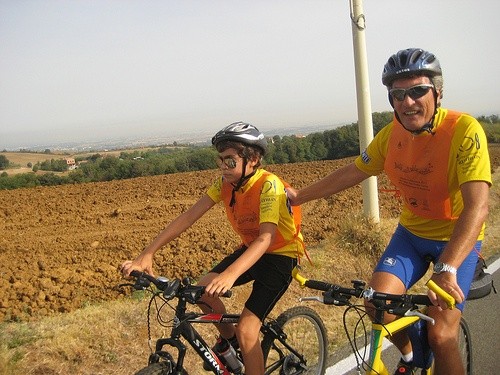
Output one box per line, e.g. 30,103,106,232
211,121,268,157
381,47,442,87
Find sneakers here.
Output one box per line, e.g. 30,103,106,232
393,357,414,375
203,334,240,371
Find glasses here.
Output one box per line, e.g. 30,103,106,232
387,83,436,101
213,154,241,168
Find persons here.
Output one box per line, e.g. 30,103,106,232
120,121,305,375
285,48,493,375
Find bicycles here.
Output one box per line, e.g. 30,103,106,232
297,277,472,375
117,266,327,375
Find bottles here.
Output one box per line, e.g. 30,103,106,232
214,334,243,373
395,365,409,375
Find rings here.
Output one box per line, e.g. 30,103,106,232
449,290,454,294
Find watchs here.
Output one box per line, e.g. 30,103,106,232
432,263,458,276
